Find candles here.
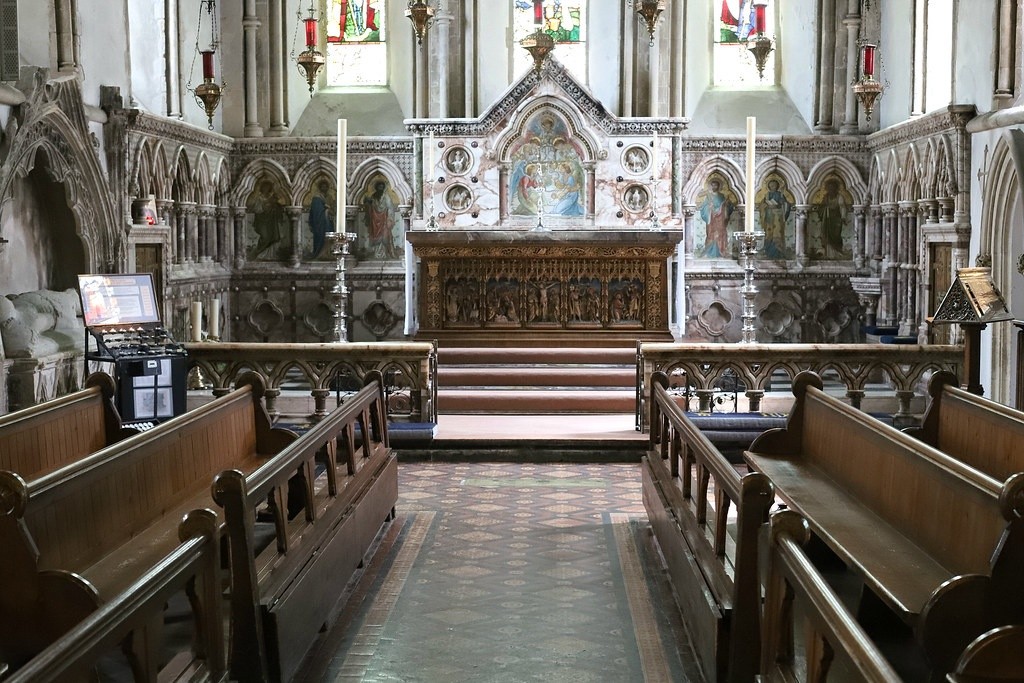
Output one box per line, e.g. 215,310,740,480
192,301,202,341
336,118,347,234
209,299,219,337
745,116,757,231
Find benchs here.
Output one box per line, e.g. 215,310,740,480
679,373,1024,683
0,371,431,683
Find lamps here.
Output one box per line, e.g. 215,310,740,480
746,0,775,79
185,0,227,130
849,0,891,122
516,0,559,82
290,0,326,99
631,0,664,47
404,0,438,50
